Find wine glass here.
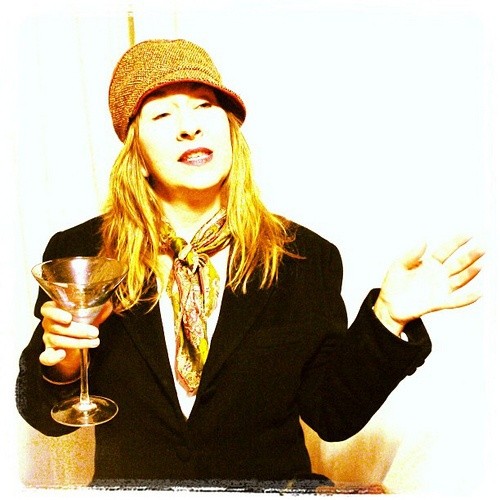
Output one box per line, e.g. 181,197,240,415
31,255,131,429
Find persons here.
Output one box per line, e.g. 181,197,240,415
14,38,486,483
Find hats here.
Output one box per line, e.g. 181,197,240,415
108,39,247,142
